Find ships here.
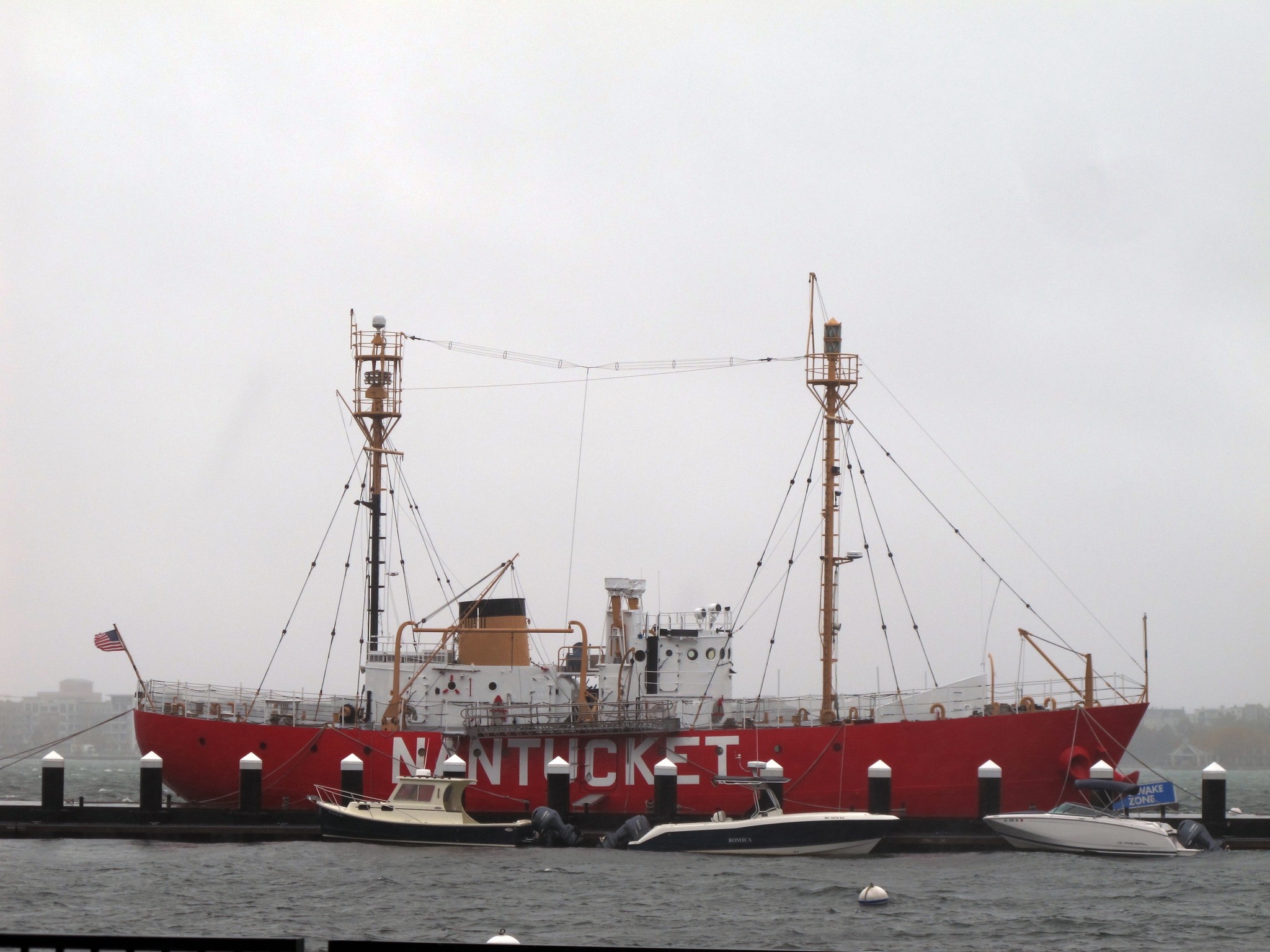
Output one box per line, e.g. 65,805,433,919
134,272,1150,821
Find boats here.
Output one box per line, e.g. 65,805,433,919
307,769,582,846
599,775,900,856
983,779,1232,857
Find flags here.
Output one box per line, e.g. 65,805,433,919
94,629,125,651
